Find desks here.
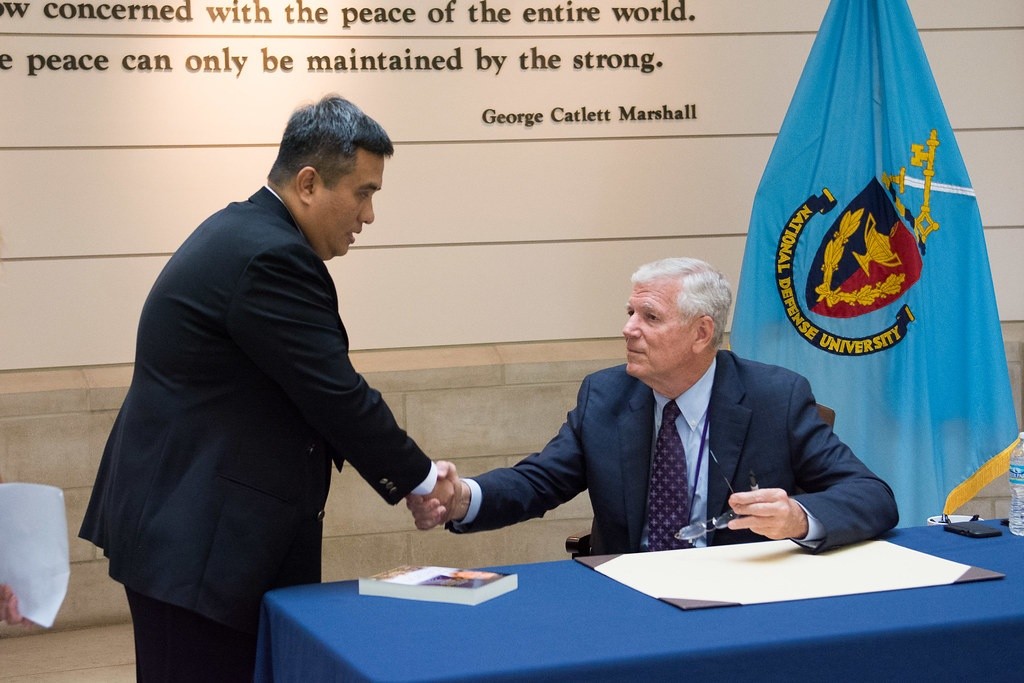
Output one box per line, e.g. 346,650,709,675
253,519,1024,683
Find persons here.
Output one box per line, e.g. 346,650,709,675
407,257,899,555
78,94,455,683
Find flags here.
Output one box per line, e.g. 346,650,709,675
729,0,1018,529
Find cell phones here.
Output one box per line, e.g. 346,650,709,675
943,521,1002,538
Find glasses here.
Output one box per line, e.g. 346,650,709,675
674,450,738,540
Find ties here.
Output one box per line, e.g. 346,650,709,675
647,401,689,551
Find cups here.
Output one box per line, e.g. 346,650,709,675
927,515,984,526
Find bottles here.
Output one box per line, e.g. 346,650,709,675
1008,432,1024,536
